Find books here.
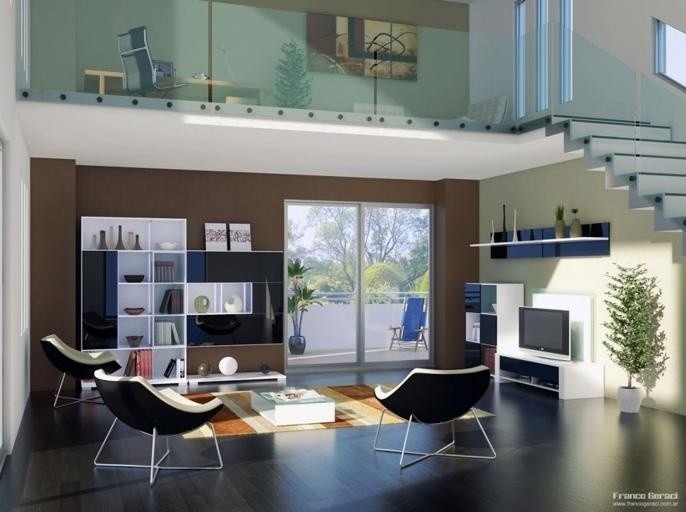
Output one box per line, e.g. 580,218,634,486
123,350,152,379
260,388,331,403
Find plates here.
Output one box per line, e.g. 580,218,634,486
219,355,238,376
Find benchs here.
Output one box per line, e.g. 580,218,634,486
451,96,508,123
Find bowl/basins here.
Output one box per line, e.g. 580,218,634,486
126,336,143,347
156,241,177,250
124,275,144,282
124,307,144,315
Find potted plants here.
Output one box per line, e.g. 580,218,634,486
287,258,324,355
603,262,663,413
569,208,583,238
553,205,565,238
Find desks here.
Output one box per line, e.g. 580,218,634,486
84,69,270,107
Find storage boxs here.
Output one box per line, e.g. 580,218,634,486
222,95,258,106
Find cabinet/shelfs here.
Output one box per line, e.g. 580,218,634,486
80,215,187,390
186,250,288,380
227,221,255,251
469,222,610,259
492,352,605,400
464,283,525,378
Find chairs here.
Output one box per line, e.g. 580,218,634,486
192,316,241,346
92,369,225,487
374,364,497,470
388,297,428,352
118,26,188,98
80,310,115,347
40,334,121,408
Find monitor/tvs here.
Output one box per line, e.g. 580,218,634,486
518,306,572,361
152,60,174,78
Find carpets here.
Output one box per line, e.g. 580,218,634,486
171,384,496,438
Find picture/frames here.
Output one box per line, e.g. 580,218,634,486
202,220,227,251
